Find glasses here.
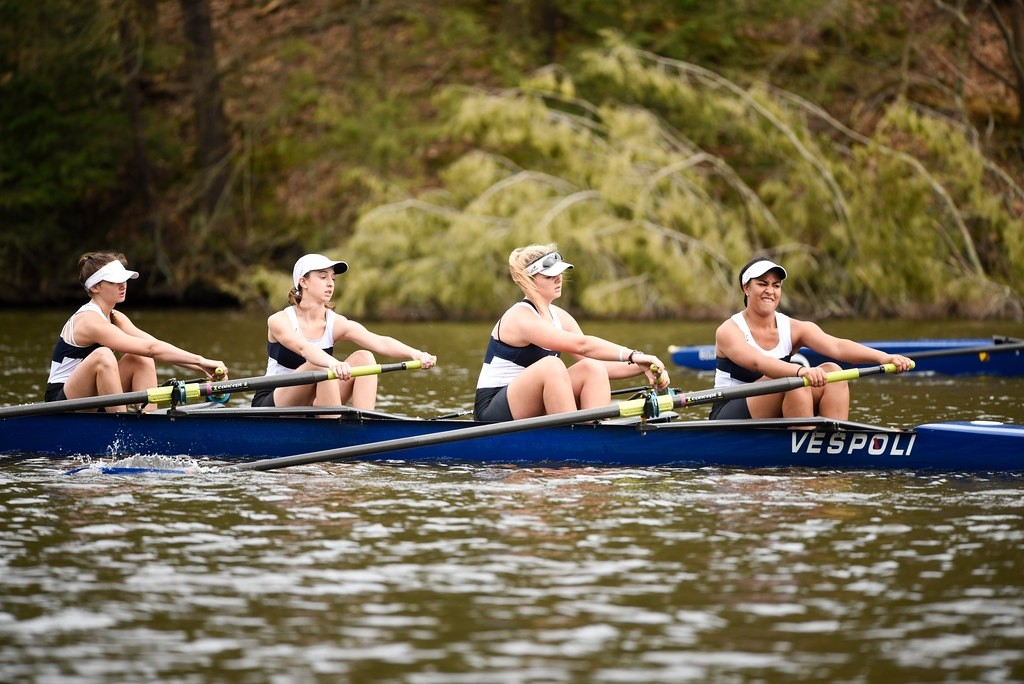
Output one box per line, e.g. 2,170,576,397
543,253,564,268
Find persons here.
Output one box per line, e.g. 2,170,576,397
44,252,229,414
710,256,912,422
473,243,671,423
250,253,437,418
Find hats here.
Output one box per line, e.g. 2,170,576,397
742,260,787,285
293,254,349,290
521,251,574,278
83,260,139,293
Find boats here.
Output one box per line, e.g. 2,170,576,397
667,334,1023,377
0,405,1024,472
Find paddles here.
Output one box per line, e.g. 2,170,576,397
1,356,438,429
205,366,231,403
648,362,682,396
64,358,917,477
